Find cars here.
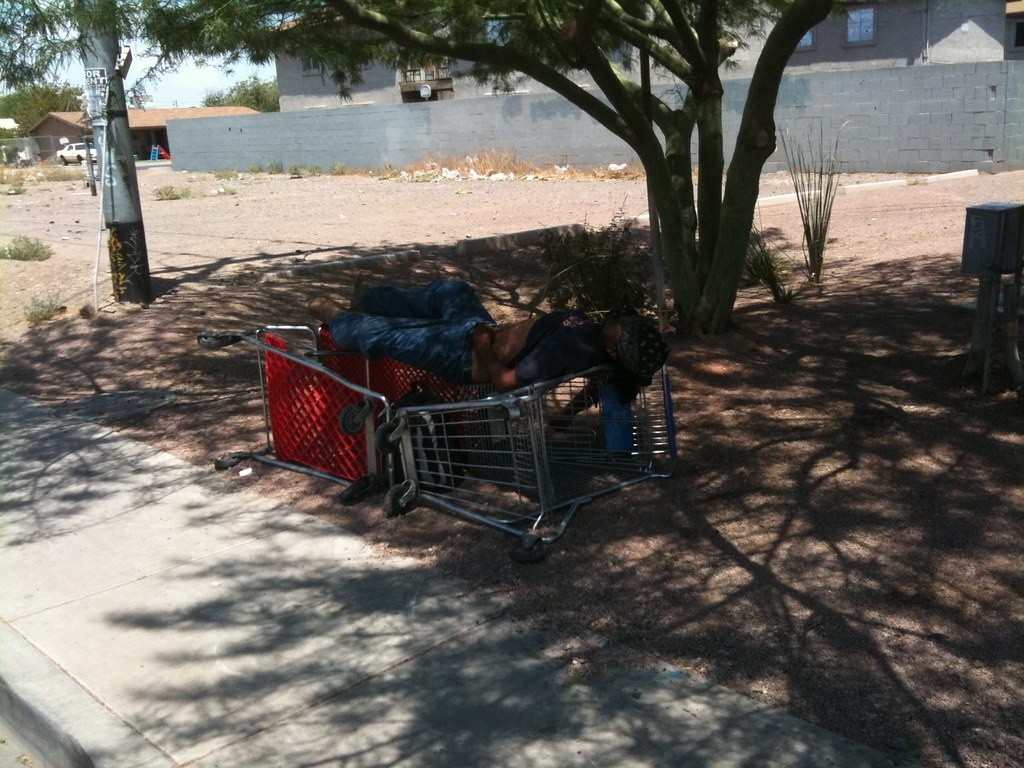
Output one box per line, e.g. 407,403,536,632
56,143,97,165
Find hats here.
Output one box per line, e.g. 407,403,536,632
617,317,671,387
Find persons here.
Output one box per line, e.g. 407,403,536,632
305,271,668,432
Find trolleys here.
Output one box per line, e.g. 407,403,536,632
196,324,681,546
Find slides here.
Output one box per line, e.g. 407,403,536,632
154,146,170,159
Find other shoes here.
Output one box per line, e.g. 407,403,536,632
308,296,346,324
353,271,377,303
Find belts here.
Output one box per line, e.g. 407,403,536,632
462,325,474,385
